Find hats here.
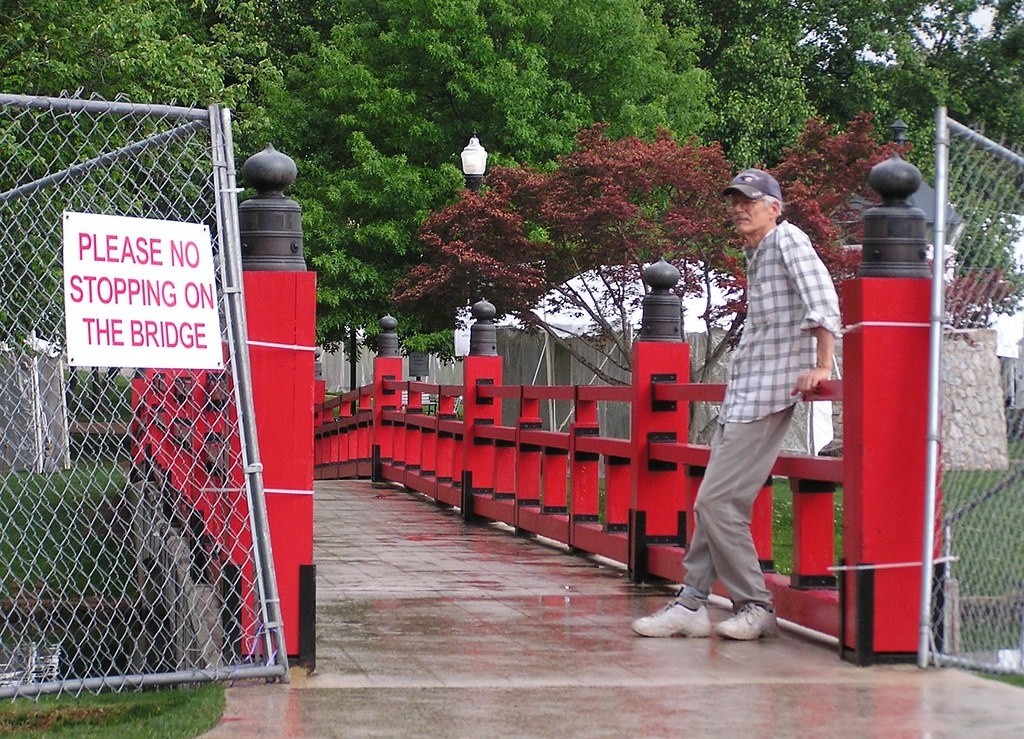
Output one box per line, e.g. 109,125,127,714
723,168,783,201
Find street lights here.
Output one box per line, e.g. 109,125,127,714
458,129,492,309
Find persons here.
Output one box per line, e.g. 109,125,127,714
631,168,840,640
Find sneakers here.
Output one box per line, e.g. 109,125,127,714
716,603,779,641
631,600,712,638
